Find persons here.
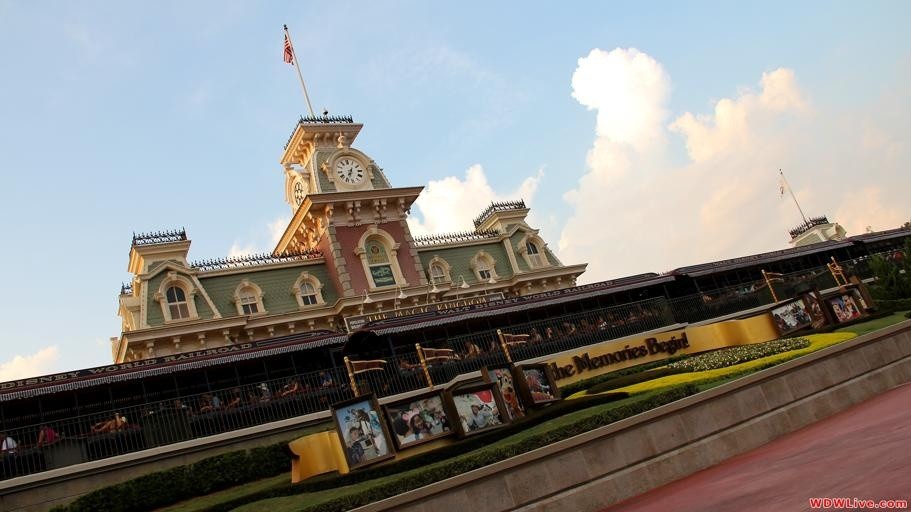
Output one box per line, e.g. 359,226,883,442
1,244,911,453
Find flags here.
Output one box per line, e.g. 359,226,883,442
780,181,787,197
283,35,294,66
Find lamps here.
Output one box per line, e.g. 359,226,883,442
394,284,408,310
457,275,470,298
426,280,441,305
484,270,497,294
359,288,374,315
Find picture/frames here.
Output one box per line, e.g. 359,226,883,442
330,389,396,471
792,288,835,330
839,283,870,314
822,290,866,323
516,362,564,409
380,388,456,449
448,382,513,440
767,294,814,336
480,362,530,424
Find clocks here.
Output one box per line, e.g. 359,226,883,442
333,156,369,189
